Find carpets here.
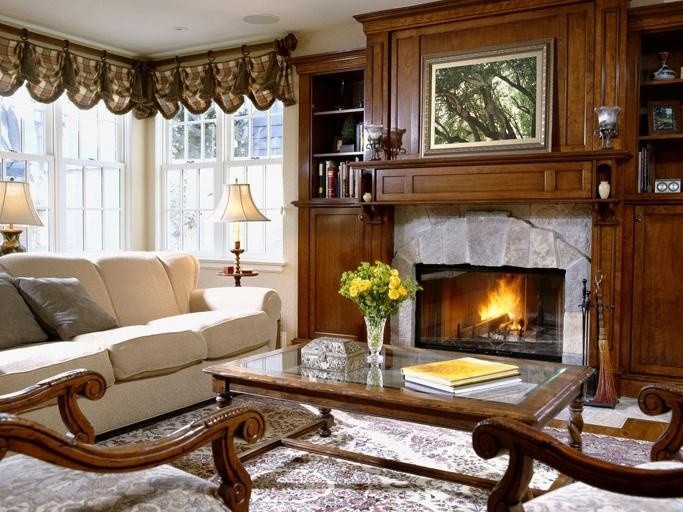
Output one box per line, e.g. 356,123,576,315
0,389,683,512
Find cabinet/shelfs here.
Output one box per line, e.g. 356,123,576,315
625,0,683,414
285,48,398,350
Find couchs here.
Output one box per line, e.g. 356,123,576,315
0,252,281,460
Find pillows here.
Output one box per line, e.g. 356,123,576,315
0,270,120,351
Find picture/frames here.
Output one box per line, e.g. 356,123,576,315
416,37,557,159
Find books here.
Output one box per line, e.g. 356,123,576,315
638,142,657,194
317,157,364,199
401,355,523,399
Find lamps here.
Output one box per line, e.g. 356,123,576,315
592,104,623,151
0,176,44,256
364,124,385,161
208,178,271,287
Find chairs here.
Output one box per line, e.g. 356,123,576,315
471,382,682,512
0,368,267,512
